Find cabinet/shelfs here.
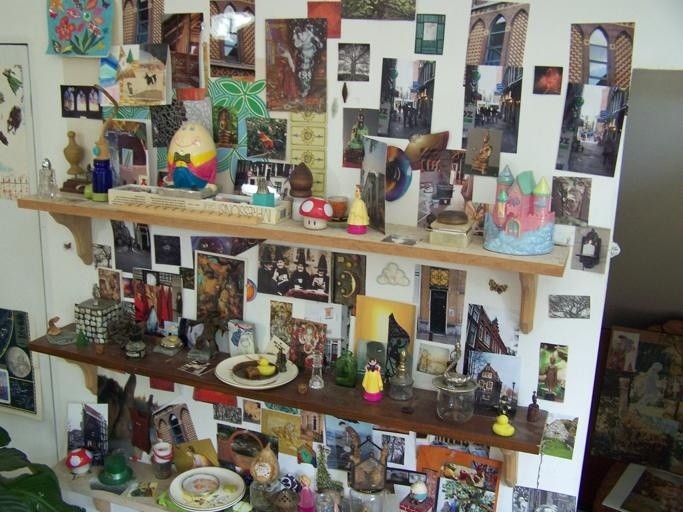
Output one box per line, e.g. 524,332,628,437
13,189,572,511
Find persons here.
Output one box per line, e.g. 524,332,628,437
339,421,361,456
545,353,557,392
480,104,500,126
257,258,328,295
395,102,418,127
363,357,385,401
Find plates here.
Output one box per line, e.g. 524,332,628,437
98,465,134,486
168,466,247,511
212,355,301,392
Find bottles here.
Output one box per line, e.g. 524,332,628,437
90,158,111,202
125,329,146,362
309,350,325,389
37,160,57,200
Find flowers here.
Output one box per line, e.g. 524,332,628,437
47,0,110,56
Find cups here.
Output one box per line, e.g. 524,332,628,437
151,443,171,479
328,196,348,220
315,493,334,512
